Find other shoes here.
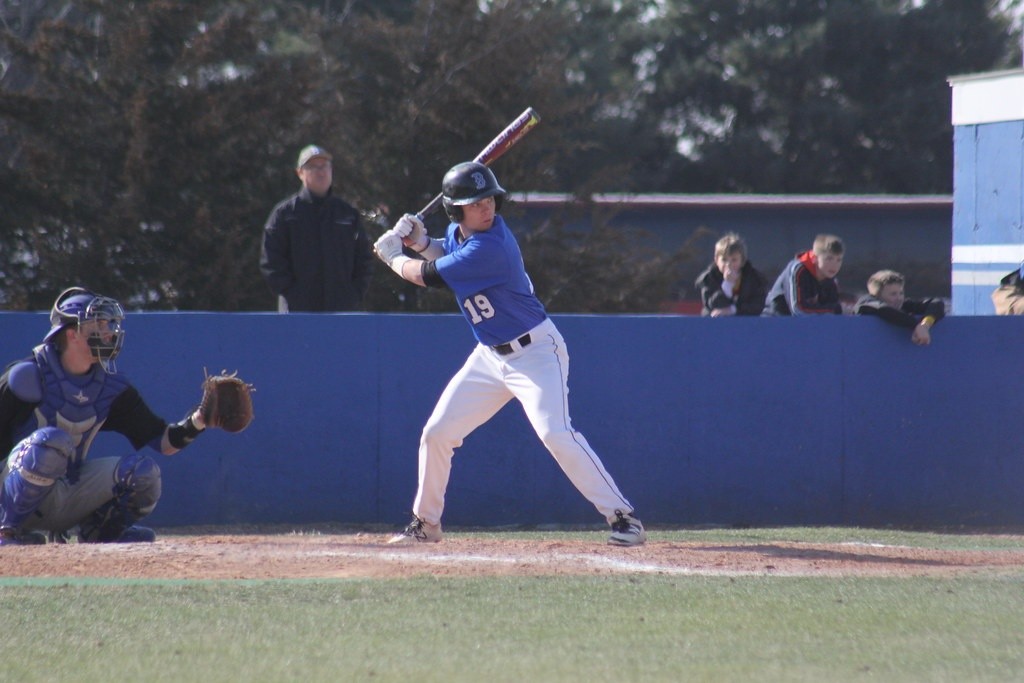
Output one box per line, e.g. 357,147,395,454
78,525,155,543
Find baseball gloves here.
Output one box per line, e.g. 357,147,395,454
195,375,255,433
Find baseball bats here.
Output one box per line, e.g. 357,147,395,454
374,104,541,251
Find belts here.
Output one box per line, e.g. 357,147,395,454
489,333,530,355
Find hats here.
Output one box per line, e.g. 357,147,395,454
298,144,331,169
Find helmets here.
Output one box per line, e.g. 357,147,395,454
43,294,112,341
441,161,505,223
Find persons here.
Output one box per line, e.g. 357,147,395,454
991,265,1024,314
852,269,945,346
760,235,845,316
374,162,650,548
696,235,766,316
260,145,380,312
0,293,252,546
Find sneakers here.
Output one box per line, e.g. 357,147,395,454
388,515,442,543
608,509,646,546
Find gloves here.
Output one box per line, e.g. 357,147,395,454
373,229,412,279
393,213,429,253
